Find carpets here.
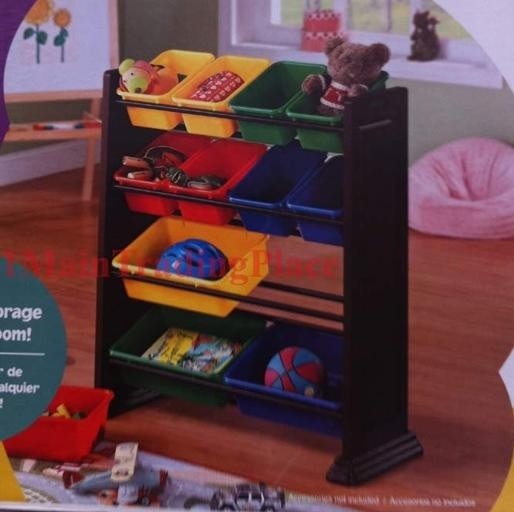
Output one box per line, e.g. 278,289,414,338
0,445,348,511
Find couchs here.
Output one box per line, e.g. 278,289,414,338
408,133,514,247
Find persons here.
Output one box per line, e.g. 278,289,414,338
117,57,182,96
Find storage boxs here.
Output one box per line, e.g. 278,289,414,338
0,378,114,467
102,42,394,441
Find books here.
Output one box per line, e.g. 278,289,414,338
142,326,246,376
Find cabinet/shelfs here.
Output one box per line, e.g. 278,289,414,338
94,57,427,488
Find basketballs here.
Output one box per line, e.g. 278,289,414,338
265,347,322,399
